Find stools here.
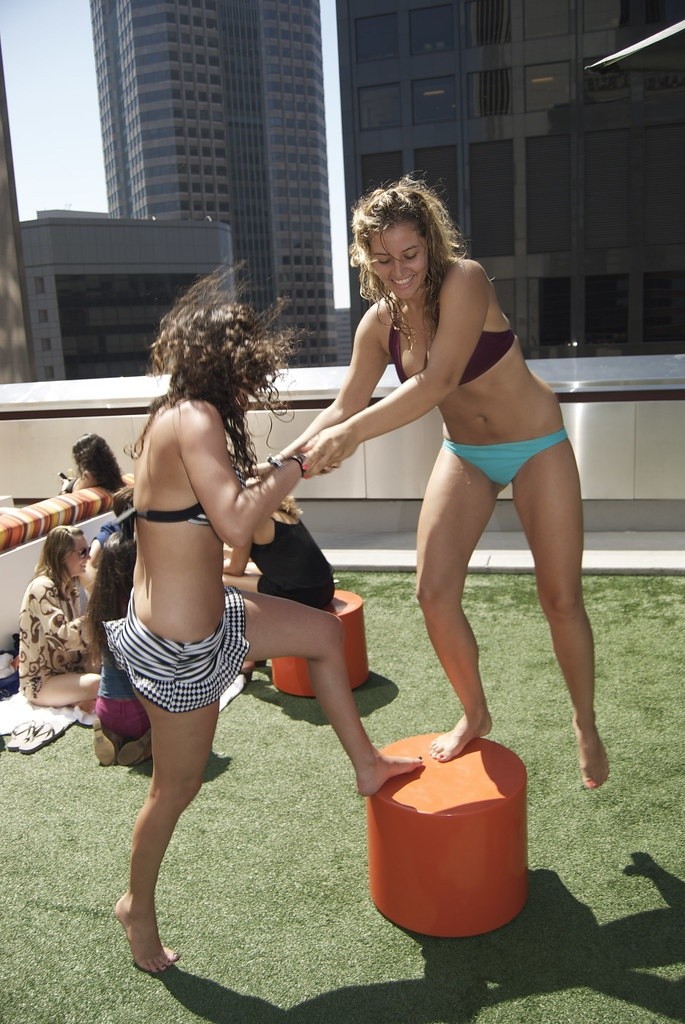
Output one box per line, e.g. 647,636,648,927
272,589,369,698
366,732,530,937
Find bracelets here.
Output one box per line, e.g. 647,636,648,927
283,454,306,478
267,453,283,469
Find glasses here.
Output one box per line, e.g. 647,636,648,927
73,546,91,559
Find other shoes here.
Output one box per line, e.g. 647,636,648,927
93,718,121,766
118,728,151,766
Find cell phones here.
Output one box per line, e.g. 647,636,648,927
59,473,71,482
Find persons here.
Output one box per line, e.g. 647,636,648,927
59,433,126,494
17,524,100,706
240,169,612,789
82,531,152,766
96,258,424,974
78,485,134,594
221,494,336,675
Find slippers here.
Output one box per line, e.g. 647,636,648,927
18,721,65,754
6,719,37,752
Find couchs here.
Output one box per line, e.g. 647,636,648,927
0,472,136,655
